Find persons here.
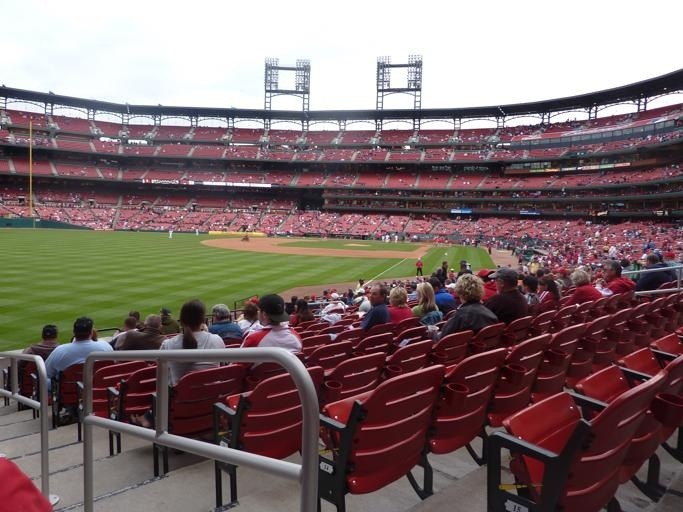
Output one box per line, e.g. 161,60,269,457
1,107,683,435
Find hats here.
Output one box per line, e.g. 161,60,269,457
256,293,289,322
356,288,364,294
243,297,259,305
488,266,518,281
